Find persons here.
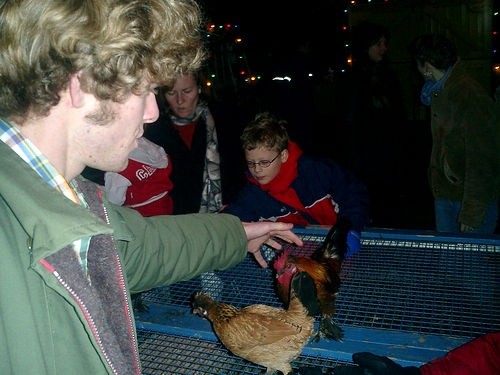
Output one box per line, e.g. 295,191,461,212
102,26,500,375
0,0,303,375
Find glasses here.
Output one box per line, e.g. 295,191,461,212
246,153,282,168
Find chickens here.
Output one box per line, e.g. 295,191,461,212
272,220,361,344
191,270,320,375
